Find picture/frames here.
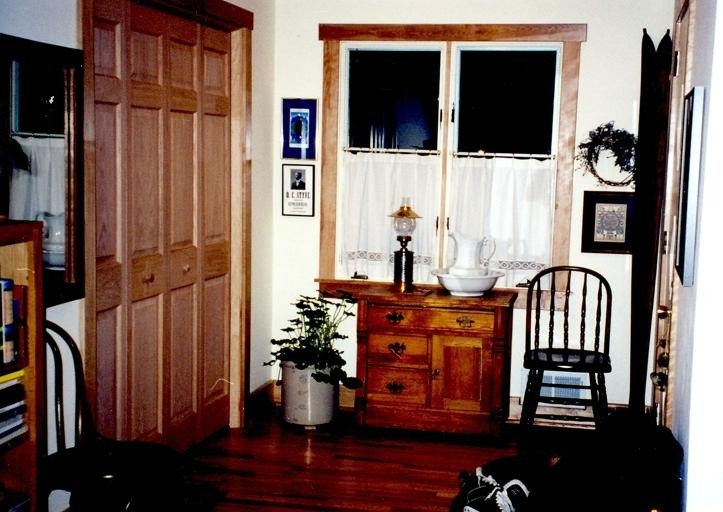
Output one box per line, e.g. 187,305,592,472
281,163,315,217
673,84,705,292
580,191,634,253
281,98,318,161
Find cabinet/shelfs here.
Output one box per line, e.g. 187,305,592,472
0,218,51,512
321,279,518,436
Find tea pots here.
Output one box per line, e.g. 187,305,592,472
36,210,69,271
445,229,496,276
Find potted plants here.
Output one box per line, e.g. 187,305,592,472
262,287,361,431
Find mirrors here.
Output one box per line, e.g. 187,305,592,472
0,34,85,310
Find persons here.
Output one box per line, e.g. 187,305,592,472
289,170,304,189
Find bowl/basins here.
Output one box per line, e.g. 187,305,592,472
436,269,505,297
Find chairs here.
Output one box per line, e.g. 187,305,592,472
44,321,176,512
516,263,612,452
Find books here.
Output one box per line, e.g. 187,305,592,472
0,276,31,512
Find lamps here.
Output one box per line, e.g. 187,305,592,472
390,197,422,285
